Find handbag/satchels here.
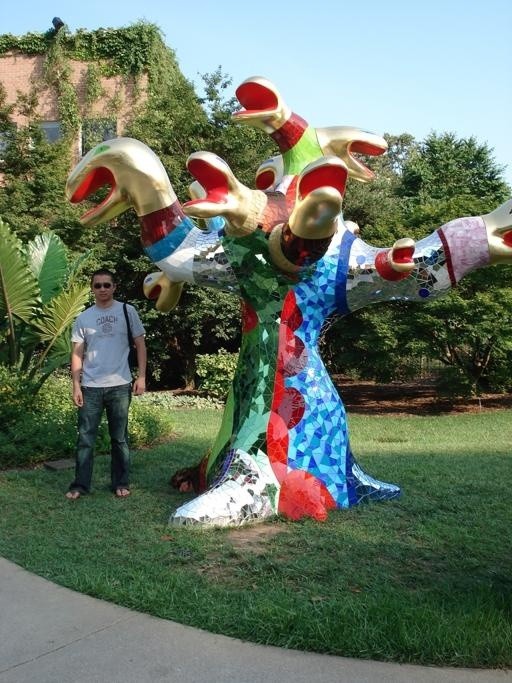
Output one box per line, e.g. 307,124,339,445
123,303,138,367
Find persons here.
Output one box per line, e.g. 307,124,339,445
65,269,148,500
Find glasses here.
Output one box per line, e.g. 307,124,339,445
93,282,111,289
